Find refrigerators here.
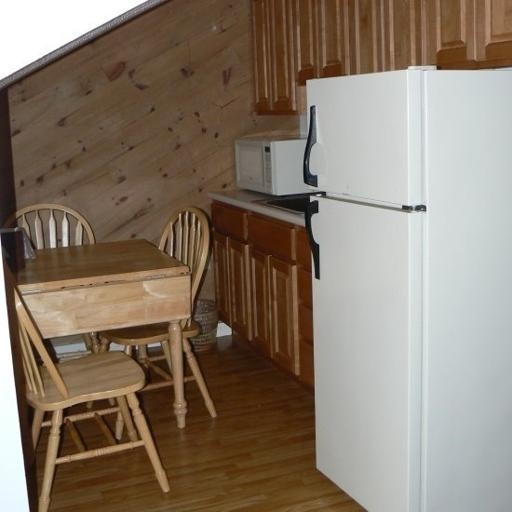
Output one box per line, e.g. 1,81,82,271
300,69,510,511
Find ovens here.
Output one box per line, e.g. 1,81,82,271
233,129,315,196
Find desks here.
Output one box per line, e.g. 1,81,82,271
4,237,197,432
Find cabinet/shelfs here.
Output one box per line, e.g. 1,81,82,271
250,2,512,116
210,200,315,395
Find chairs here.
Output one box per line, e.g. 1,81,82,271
4,264,174,512
83,204,218,439
2,204,99,357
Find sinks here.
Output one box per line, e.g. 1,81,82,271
254,192,306,215
